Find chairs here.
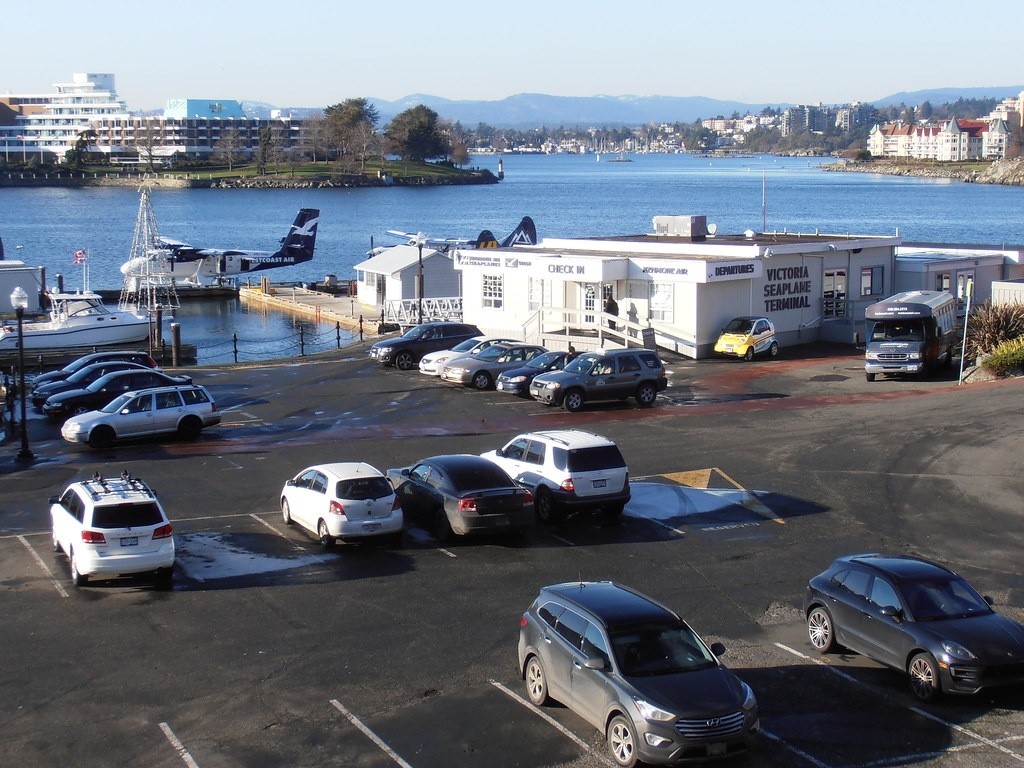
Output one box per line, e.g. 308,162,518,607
505,352,514,362
142,398,151,411
515,350,523,361
164,394,180,406
432,328,440,339
594,361,614,373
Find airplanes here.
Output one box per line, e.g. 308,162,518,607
365,215,537,262
120,208,320,288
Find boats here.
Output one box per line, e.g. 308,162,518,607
0,248,175,350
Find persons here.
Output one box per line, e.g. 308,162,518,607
605,296,619,335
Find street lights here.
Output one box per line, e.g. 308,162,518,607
10,283,35,458
414,231,426,324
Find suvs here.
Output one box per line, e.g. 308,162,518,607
47,477,176,587
529,347,668,413
31,351,159,388
369,321,491,371
480,427,632,526
518,578,760,768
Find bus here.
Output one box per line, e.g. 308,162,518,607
853,290,958,382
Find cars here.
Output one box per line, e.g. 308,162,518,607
31,359,164,407
418,335,526,377
61,384,222,449
386,454,537,544
713,316,779,362
280,461,404,549
440,342,550,391
495,350,595,400
801,553,1024,703
42,369,193,419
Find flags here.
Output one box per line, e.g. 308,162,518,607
74,249,86,264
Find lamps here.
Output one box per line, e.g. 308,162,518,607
448,249,453,259
828,244,834,252
763,247,771,258
